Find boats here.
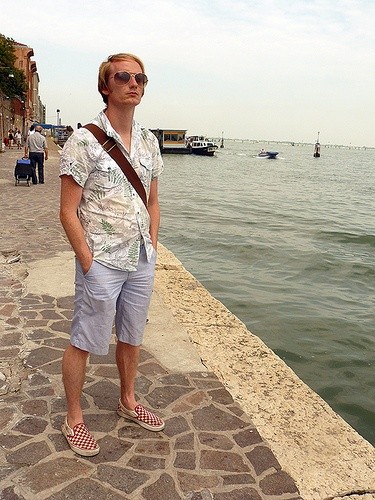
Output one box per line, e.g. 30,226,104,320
185,135,218,156
255,151,279,159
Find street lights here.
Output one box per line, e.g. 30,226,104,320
0,66,15,78
6,95,25,104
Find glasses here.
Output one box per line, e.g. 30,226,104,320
109,71,148,89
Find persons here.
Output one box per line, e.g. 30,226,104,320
4,125,66,150
24,125,49,184
166,136,193,145
57,52,165,457
260,149,263,154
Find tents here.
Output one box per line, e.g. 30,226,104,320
52,125,65,133
33,123,53,135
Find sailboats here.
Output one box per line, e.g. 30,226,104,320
313,132,321,157
220,131,225,148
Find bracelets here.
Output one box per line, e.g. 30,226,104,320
25,154,27,156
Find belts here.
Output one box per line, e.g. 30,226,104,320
140,238,145,246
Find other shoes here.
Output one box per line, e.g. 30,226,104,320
39,182,44,184
61,416,101,457
117,398,165,432
33,181,37,184
